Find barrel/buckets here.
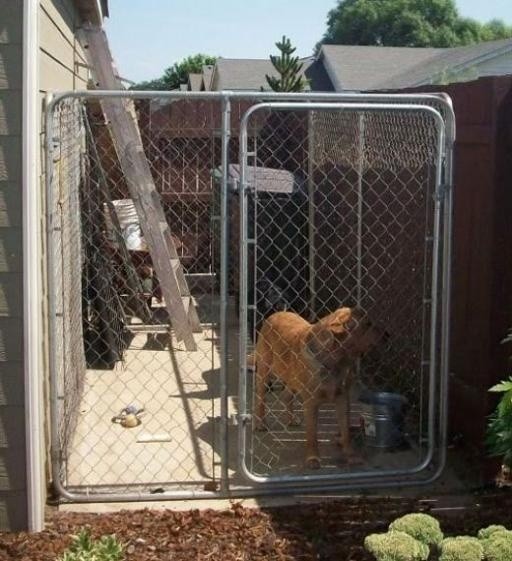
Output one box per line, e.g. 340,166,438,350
358,392,412,453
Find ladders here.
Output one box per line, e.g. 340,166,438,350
82,27,205,350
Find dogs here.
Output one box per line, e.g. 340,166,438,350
253,307,391,471
